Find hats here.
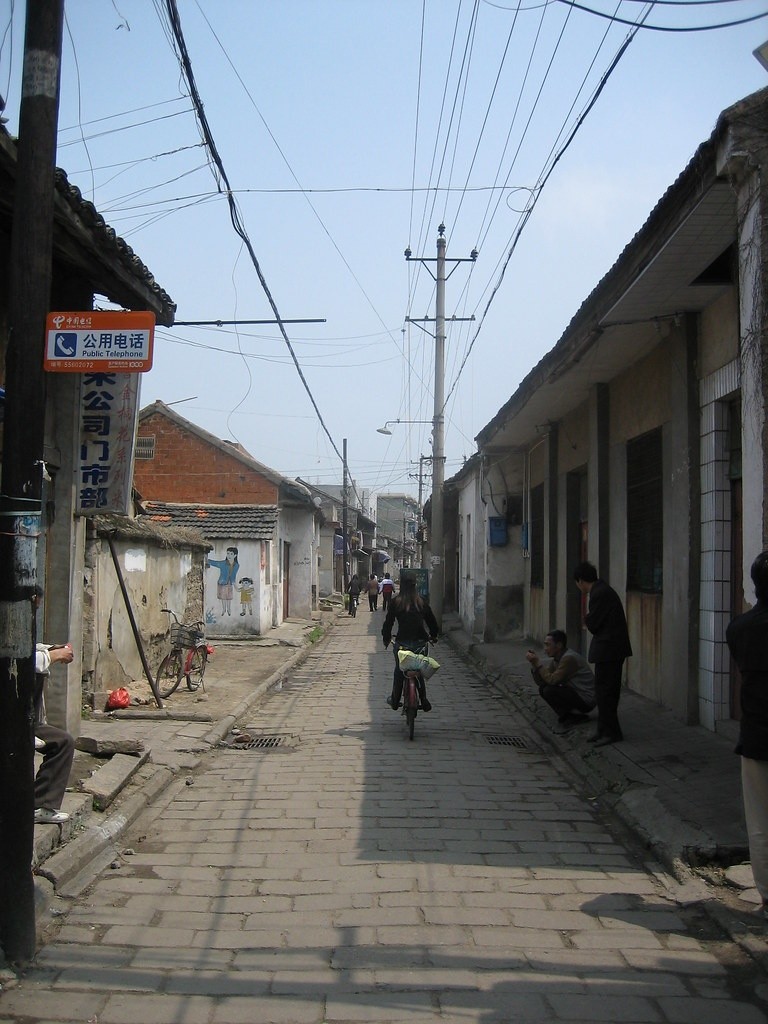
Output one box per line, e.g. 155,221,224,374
401,571,416,584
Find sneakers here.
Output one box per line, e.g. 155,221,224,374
34,807,70,823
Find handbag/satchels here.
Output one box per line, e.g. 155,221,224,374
396,649,439,679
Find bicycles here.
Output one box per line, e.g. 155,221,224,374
155,608,210,699
384,634,436,741
347,591,360,618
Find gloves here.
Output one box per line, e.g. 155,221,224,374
431,636,439,644
382,634,391,647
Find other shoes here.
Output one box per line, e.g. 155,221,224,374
559,711,588,722
388,694,399,710
374,606,378,611
587,730,621,746
420,697,431,713
348,612,351,615
357,603,360,605
370,609,373,612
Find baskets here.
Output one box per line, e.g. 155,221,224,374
170,623,196,649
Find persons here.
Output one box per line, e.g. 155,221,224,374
526,630,596,734
374,574,377,581
364,574,379,611
378,573,395,610
32,584,75,823
571,562,634,747
347,574,361,615
725,551,768,920
381,574,439,712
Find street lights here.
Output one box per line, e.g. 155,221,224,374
376,420,445,637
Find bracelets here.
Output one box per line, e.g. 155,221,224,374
537,665,542,670
